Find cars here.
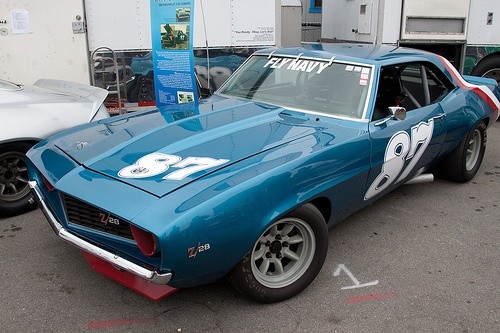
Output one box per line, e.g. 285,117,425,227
0,79,111,218
24,41,500,313
128,48,268,105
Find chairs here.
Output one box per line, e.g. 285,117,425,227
309,70,338,96
373,68,405,120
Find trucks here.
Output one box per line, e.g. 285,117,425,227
320,0,500,119
